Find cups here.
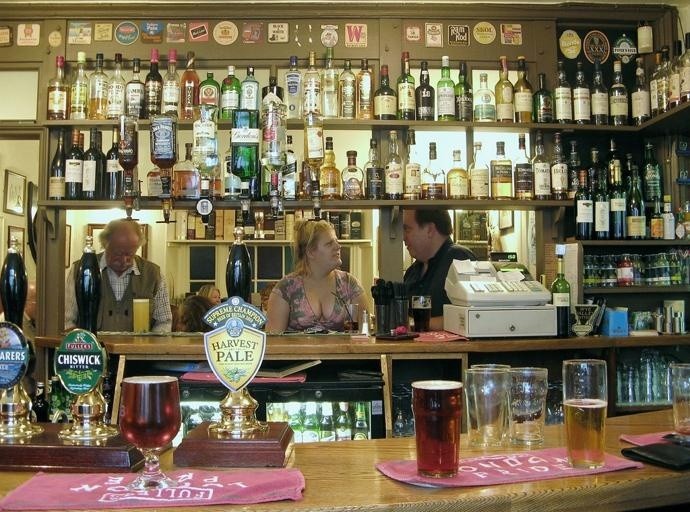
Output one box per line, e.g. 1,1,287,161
409,378,465,480
130,296,153,334
338,295,435,341
463,362,547,452
561,356,611,470
615,349,681,409
670,362,689,435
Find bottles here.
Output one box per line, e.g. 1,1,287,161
584,249,690,336
47,30,690,239
31,369,112,423
269,401,373,444
550,253,572,340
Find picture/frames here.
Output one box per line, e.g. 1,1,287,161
8,226,25,262
87,224,148,259
3,169,27,217
65,224,71,269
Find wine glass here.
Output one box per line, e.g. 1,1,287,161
113,374,186,491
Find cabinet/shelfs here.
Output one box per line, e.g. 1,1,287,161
38,102,690,356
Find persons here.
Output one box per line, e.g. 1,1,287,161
63,219,174,332
0,283,37,397
267,218,370,333
176,295,216,333
198,285,222,306
402,208,480,332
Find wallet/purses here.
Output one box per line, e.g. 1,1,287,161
621,443,689,471
